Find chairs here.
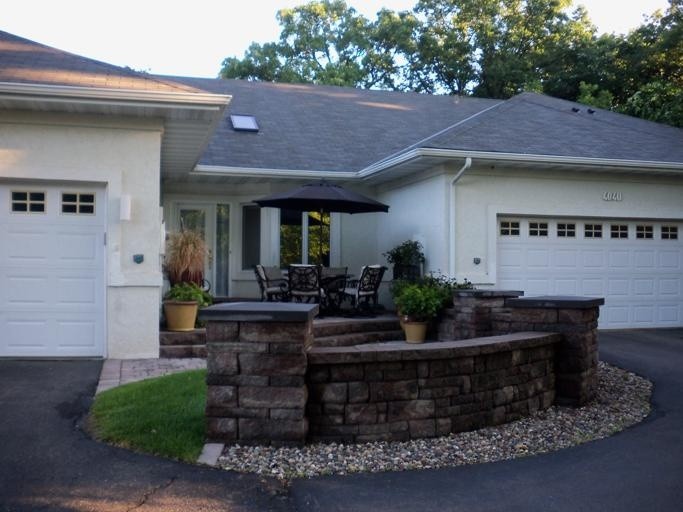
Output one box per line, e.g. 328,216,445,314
251,264,385,317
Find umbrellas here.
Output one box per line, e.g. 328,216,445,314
252,176,390,266
277,209,333,226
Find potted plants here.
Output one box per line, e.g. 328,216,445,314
382,239,426,280
162,281,211,332
389,275,451,344
